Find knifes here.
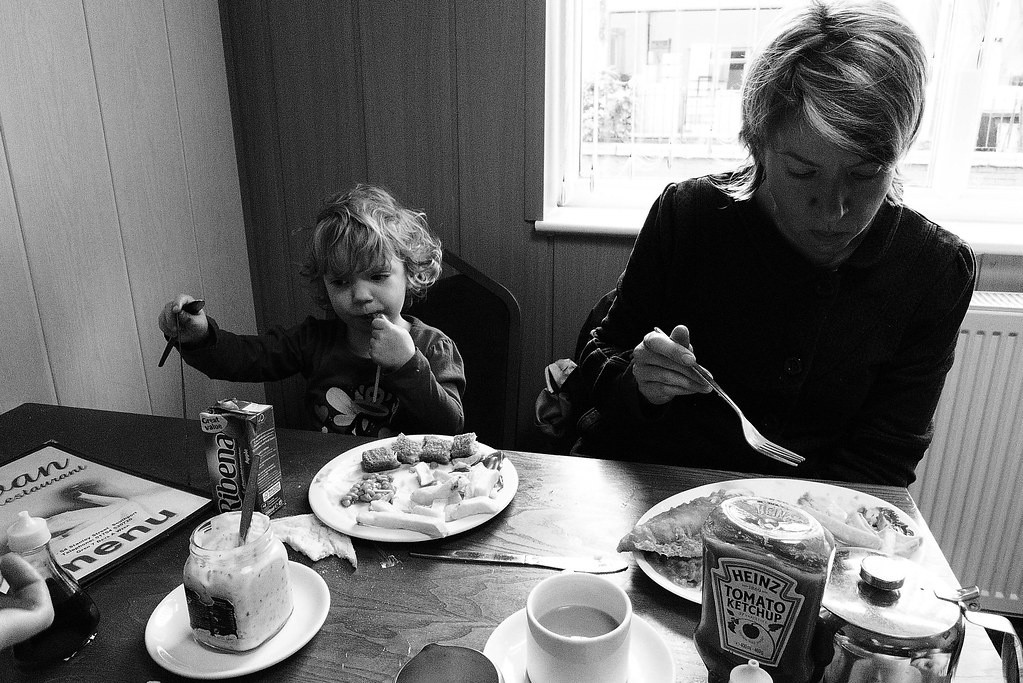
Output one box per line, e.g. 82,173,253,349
407,548,628,574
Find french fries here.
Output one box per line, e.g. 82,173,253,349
793,492,897,553
354,461,500,538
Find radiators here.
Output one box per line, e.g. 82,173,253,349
908,303,1023,615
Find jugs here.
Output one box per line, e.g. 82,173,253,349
803,547,1023,683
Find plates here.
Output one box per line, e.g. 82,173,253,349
633,478,925,607
482,608,676,683
309,435,519,545
143,560,330,678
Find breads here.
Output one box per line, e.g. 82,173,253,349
268,514,357,569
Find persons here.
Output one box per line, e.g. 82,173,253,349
556,0,977,487
159,184,465,438
0,552,55,648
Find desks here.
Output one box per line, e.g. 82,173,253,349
0,403,1004,683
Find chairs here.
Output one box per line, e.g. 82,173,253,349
325,249,523,450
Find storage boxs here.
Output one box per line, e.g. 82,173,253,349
199,398,286,517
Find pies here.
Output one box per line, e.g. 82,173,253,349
617,489,749,558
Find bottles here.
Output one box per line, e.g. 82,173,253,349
7,511,101,676
729,659,773,683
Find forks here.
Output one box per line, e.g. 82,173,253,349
653,327,806,466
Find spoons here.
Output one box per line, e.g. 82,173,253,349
158,298,205,372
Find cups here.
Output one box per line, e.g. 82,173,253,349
526,572,633,683
